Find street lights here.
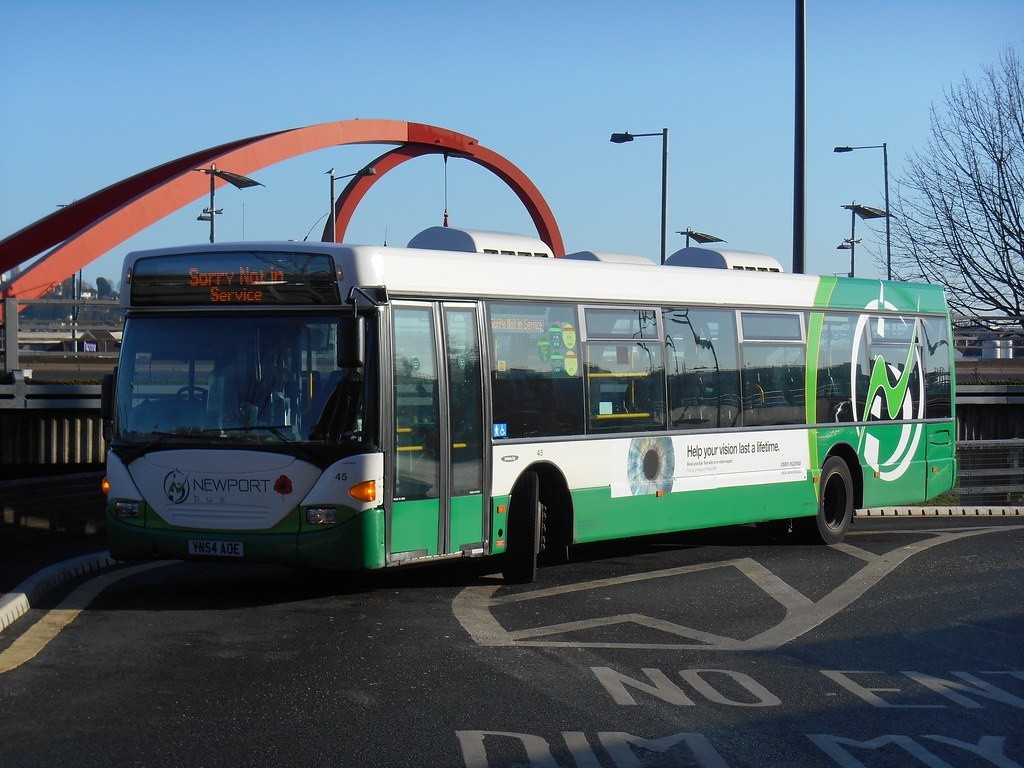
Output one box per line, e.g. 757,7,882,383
834,143,892,280
56,204,82,353
330,167,377,243
609,128,668,265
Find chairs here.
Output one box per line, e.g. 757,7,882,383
176,386,208,427
648,386,856,427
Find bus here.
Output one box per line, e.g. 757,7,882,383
102,242,959,584
